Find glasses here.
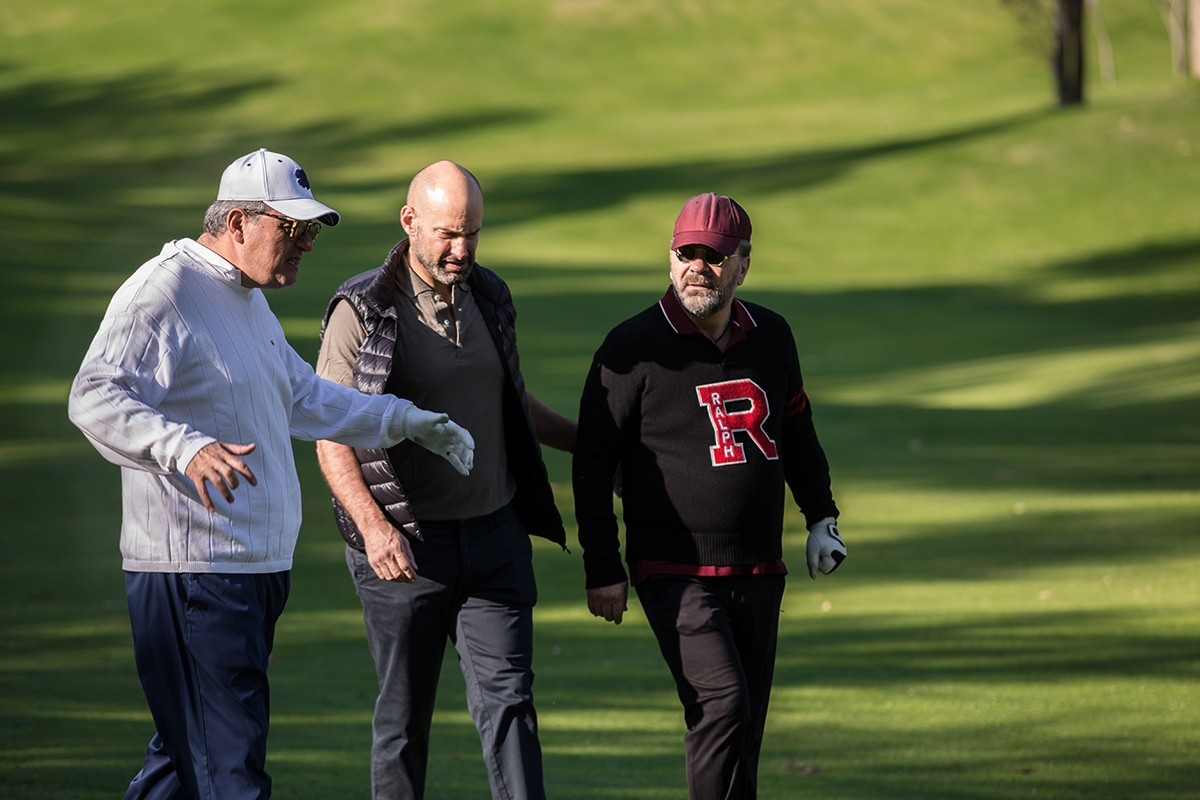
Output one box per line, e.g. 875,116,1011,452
672,244,746,267
243,209,321,244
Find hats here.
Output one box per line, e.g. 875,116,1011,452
217,148,341,228
671,192,752,258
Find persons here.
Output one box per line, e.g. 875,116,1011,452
313,159,624,800
573,192,848,799
66,145,476,800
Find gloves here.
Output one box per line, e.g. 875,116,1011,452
402,406,475,477
806,517,847,580
